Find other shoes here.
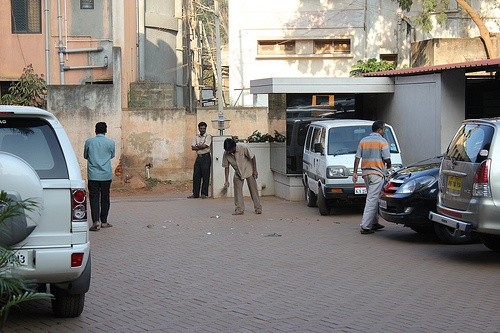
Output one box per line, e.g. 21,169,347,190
360,227,375,233
372,222,385,229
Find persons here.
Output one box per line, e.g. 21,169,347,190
352,120,392,234
221,137,262,215
83,122,115,230
186,122,212,199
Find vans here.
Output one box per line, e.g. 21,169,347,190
429,118,499,249
286,117,339,175
301,119,403,216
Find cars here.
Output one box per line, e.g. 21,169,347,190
377,153,442,237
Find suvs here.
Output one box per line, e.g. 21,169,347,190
0,102,92,318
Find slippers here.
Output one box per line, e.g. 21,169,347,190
101,222,112,228
89,225,100,231
